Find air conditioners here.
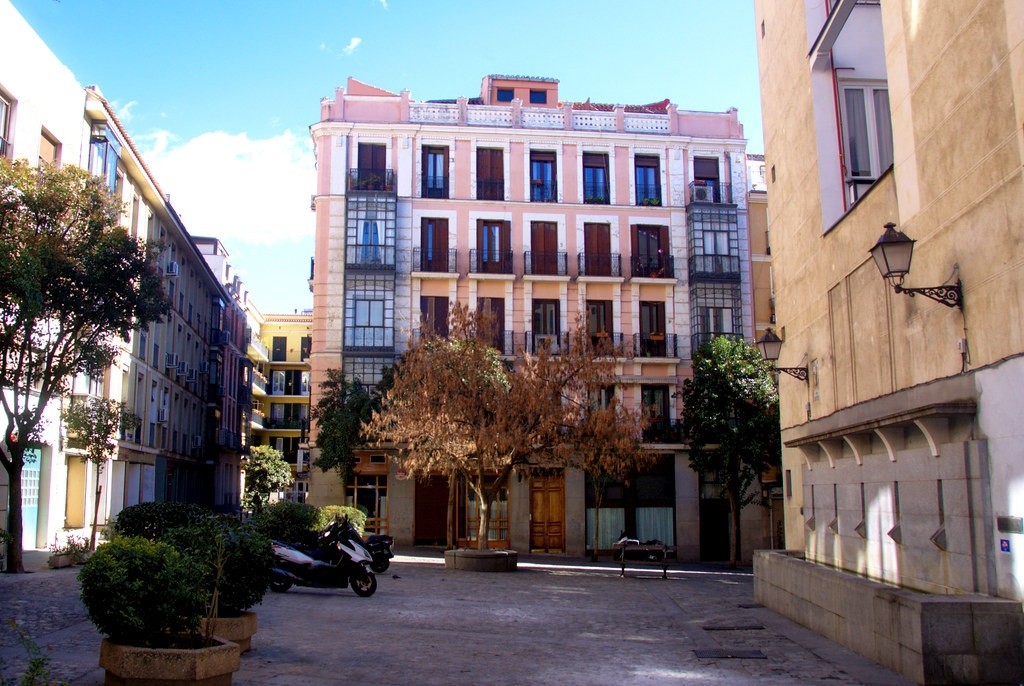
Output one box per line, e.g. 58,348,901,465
160,408,169,424
689,180,715,203
163,260,179,278
191,435,202,450
187,367,199,383
165,352,180,370
201,360,211,375
177,360,190,376
221,330,231,346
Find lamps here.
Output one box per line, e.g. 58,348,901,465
867,222,965,310
758,325,812,384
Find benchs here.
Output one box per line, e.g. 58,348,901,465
612,539,677,580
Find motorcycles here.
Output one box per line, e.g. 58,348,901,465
340,515,396,573
269,515,377,596
